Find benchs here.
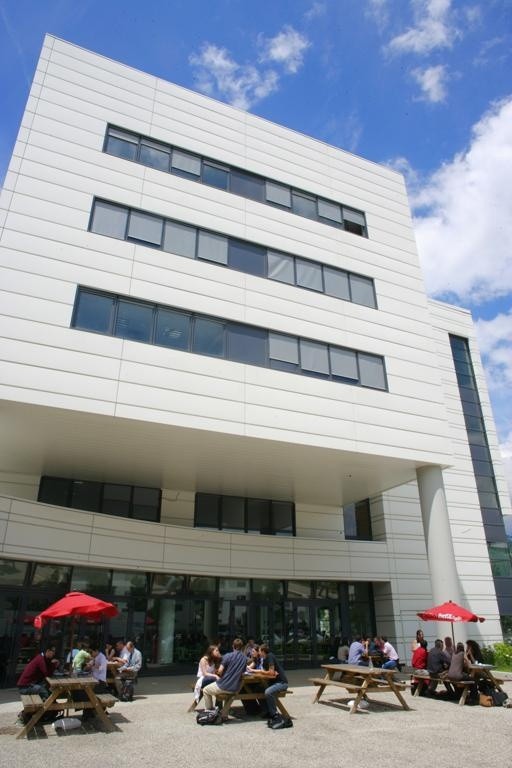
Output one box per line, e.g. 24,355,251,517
487,677,512,684
413,675,474,687
346,675,413,691
96,694,118,707
309,678,365,693
277,690,293,697
191,684,227,701
19,694,43,712
396,663,405,667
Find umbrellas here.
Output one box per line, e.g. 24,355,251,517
33,589,121,662
414,598,487,648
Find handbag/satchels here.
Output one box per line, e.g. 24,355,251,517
197,706,220,725
478,695,494,706
493,691,508,706
479,679,495,695
468,692,480,705
268,714,293,729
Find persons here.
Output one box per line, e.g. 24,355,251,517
17,634,145,722
337,633,400,672
410,629,487,695
191,632,299,729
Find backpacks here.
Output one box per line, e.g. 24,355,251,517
119,677,134,702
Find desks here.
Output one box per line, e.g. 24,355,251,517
190,670,289,722
14,677,116,738
414,664,503,706
313,664,409,714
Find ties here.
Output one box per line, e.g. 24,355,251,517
127,652,133,668
